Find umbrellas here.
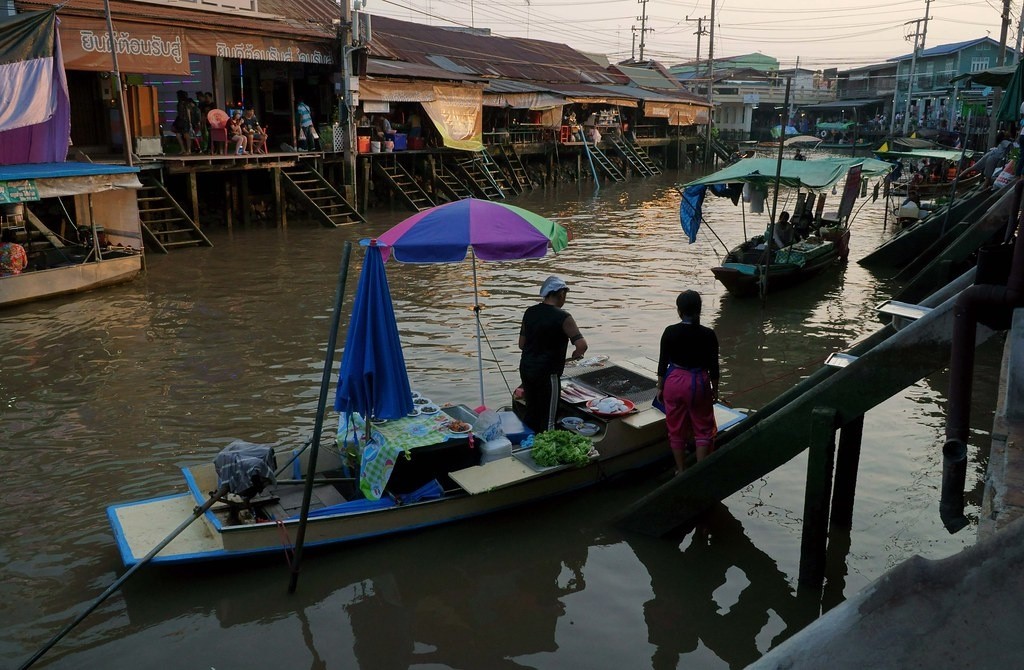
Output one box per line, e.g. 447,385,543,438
359,194,574,405
208,109,228,128
334,240,414,443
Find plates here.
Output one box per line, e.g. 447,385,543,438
364,415,387,424
449,423,473,434
406,391,440,416
585,398,635,415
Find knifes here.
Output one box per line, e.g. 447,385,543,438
566,356,584,362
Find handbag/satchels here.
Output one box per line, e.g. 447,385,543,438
171,122,184,133
300,128,306,140
309,126,319,139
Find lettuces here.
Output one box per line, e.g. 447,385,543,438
531,429,594,467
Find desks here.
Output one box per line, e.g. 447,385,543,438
336,396,477,503
358,125,376,136
775,240,834,267
561,125,568,143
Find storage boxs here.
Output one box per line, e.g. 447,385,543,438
409,137,425,149
386,133,408,151
358,135,371,153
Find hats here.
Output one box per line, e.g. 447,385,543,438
540,275,569,299
298,98,304,102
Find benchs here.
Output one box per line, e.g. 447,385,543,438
208,108,267,156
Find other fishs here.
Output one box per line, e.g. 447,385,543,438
561,381,597,403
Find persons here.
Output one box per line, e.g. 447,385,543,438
170,90,201,156
946,166,957,182
407,111,421,138
519,277,588,435
296,95,316,151
772,211,793,251
873,111,925,131
730,150,742,162
240,106,268,155
587,126,601,147
902,192,921,208
226,110,250,155
657,290,721,478
196,92,217,155
377,115,391,139
793,203,813,242
355,115,370,127
793,149,803,161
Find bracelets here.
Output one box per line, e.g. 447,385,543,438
657,382,663,389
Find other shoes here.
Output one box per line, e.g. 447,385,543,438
298,148,307,151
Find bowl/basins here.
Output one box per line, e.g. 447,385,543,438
562,417,584,429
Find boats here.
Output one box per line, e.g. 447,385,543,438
875,150,982,197
106,354,746,569
674,157,882,295
729,141,757,161
770,125,823,162
810,122,873,150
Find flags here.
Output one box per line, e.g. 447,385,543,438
953,135,961,150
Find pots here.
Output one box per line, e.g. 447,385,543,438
575,423,600,437
